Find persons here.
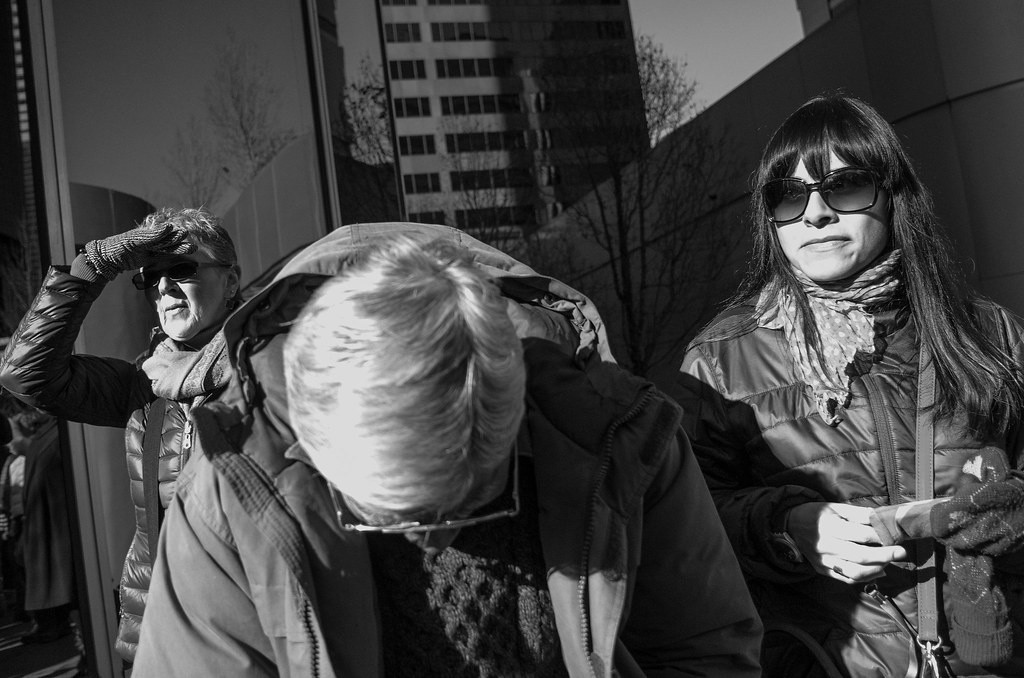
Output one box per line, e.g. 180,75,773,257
130,221,766,678
0,206,246,678
672,98,1024,678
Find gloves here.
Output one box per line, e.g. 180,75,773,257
85,224,199,280
938,476,1024,558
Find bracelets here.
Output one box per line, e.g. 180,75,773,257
79,249,101,275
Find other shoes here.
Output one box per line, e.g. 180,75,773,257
19,619,76,643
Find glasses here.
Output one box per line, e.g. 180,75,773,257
759,165,885,225
328,440,522,535
131,262,233,290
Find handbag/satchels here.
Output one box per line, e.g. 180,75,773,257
761,575,955,678
